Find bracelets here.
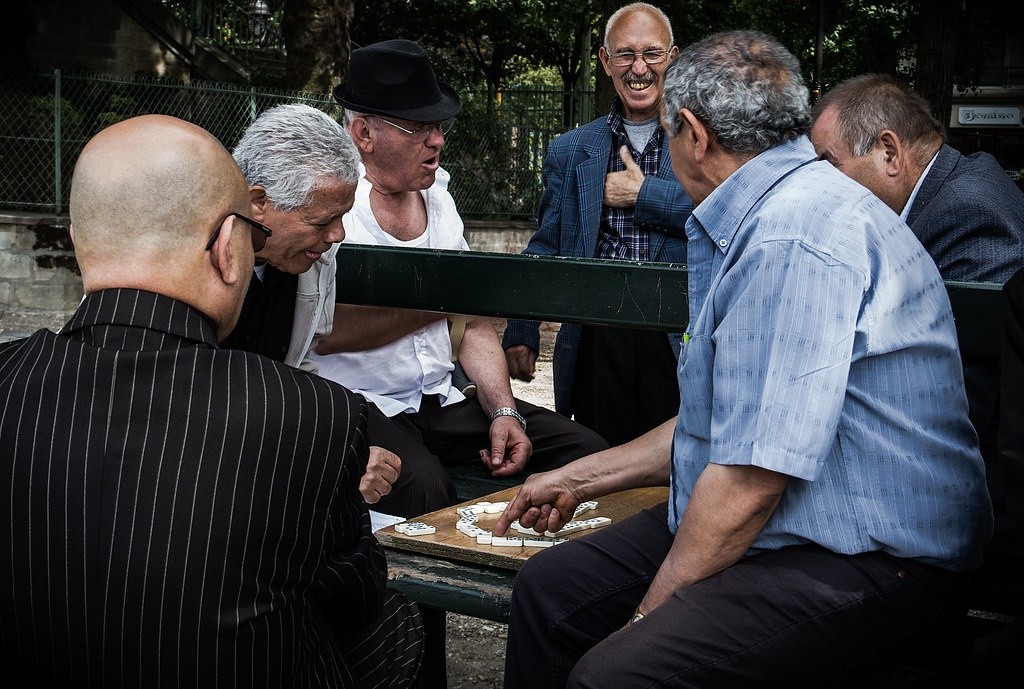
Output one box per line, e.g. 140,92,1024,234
493,407,526,432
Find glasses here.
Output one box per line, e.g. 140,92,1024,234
363,114,457,144
204,210,273,253
605,49,671,66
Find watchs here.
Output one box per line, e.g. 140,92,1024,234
631,607,644,623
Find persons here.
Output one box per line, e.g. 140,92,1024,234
492,28,993,689
811,71,1024,607
230,105,403,505
306,40,609,491
502,1,700,448
0,113,425,688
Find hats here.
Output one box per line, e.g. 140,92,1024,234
331,39,463,122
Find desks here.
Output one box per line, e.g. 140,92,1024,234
374,484,670,689
